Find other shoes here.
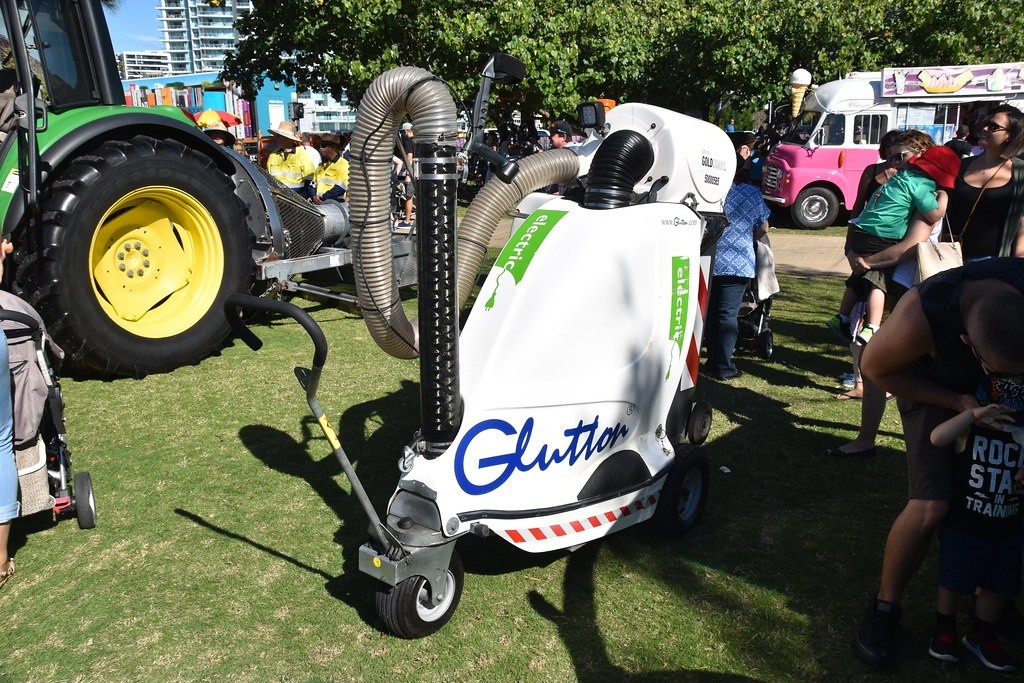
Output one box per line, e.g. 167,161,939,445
824,446,877,458
0,558,14,587
721,370,742,380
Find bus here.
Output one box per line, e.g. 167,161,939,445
761,60,1024,230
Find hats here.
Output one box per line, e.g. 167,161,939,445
267,121,303,142
549,119,571,137
203,122,235,147
908,145,961,188
976,372,1024,427
399,123,413,130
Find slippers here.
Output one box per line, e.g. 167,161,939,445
836,394,863,399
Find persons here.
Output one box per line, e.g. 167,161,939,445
824,103,1024,672
459,120,583,196
0,328,21,588
201,120,418,229
726,118,764,180
704,160,771,381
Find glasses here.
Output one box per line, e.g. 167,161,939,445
970,344,1024,378
552,123,566,133
886,150,924,164
983,120,1010,131
320,143,334,148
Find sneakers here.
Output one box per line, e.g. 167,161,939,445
856,327,874,345
961,626,1017,671
857,596,904,666
826,314,853,341
396,220,411,228
928,622,959,661
966,595,1024,643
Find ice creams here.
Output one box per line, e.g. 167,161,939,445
790,68,811,117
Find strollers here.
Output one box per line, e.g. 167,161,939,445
0,305,97,529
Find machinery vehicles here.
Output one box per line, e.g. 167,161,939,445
0,1,418,382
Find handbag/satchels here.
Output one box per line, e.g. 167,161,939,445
943,234,964,250
914,211,963,284
397,169,406,181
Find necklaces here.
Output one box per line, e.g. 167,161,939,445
981,156,995,174
